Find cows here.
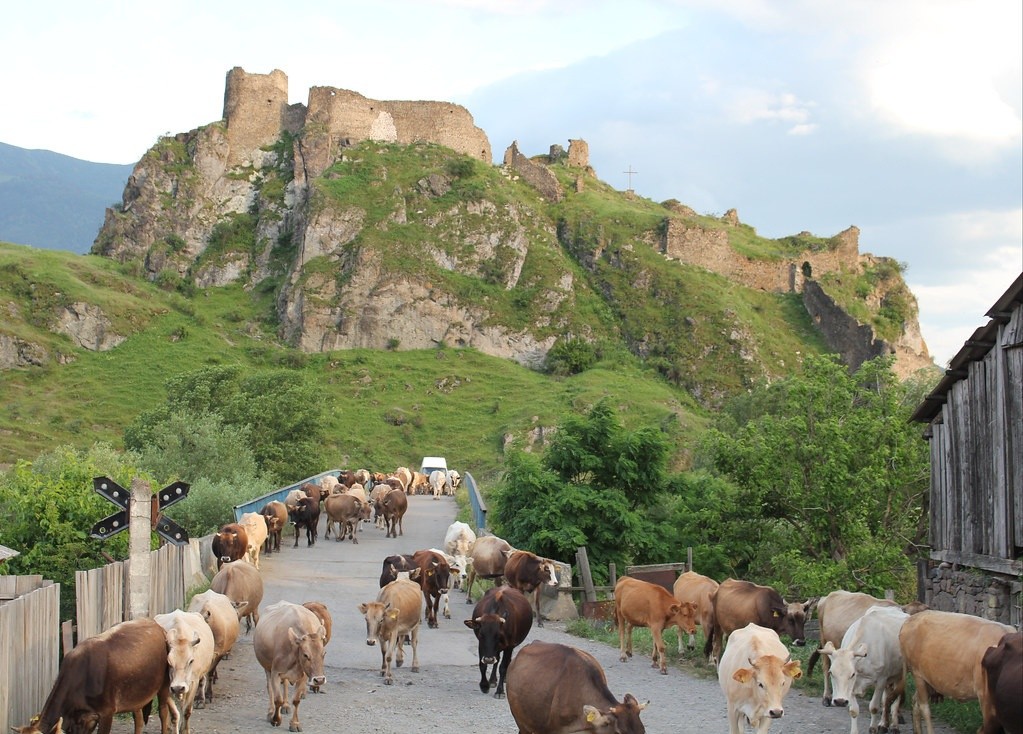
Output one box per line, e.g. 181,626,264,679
506,640,655,734
805,588,928,681
155,609,214,734
212,511,268,571
899,609,1023,734
184,589,248,704
375,490,408,539
12,615,179,734
260,499,289,553
284,467,461,516
611,572,816,676
816,608,912,734
324,494,369,543
288,497,320,548
252,600,332,733
715,621,801,734
358,549,473,684
444,520,561,628
205,558,264,635
463,582,533,700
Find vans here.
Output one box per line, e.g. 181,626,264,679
419,456,450,477
